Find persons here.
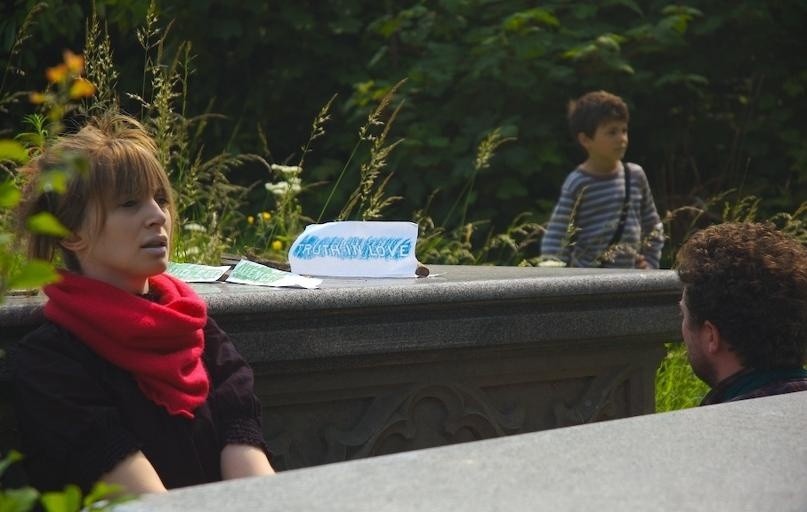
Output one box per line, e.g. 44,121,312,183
0,108,277,500
540,89,665,270
675,217,806,406
